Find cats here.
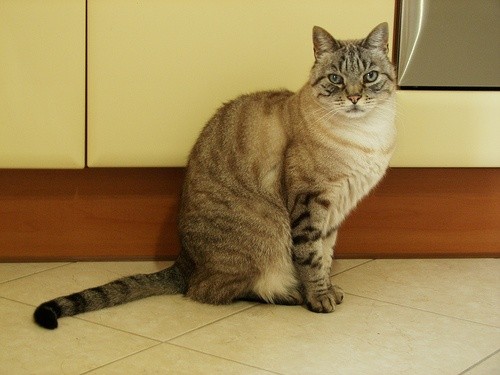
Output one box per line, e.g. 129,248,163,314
33,21,398,330
